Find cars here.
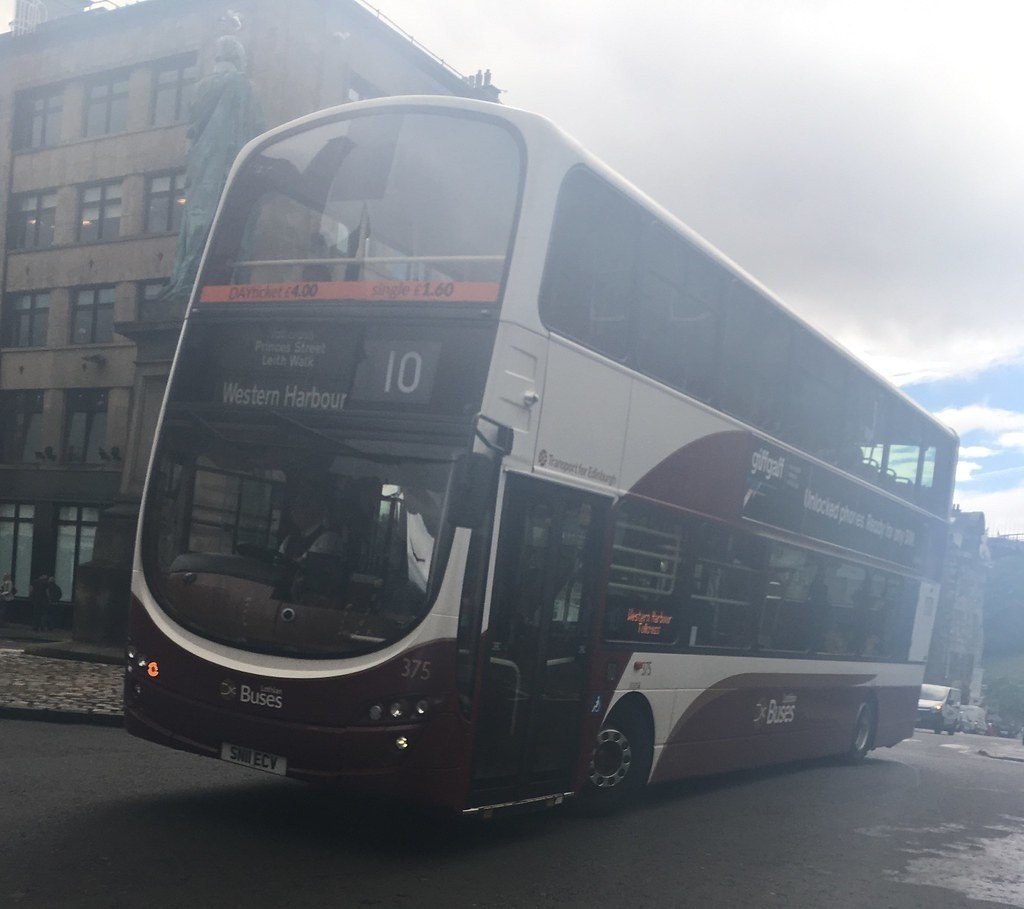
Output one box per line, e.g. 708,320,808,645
960,703,1020,736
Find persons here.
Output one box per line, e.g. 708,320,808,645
276,494,331,561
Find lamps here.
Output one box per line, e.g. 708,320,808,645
80,353,106,364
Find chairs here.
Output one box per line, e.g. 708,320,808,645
275,499,370,613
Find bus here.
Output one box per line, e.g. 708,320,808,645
100,92,962,819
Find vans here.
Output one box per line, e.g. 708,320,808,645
917,685,961,736
